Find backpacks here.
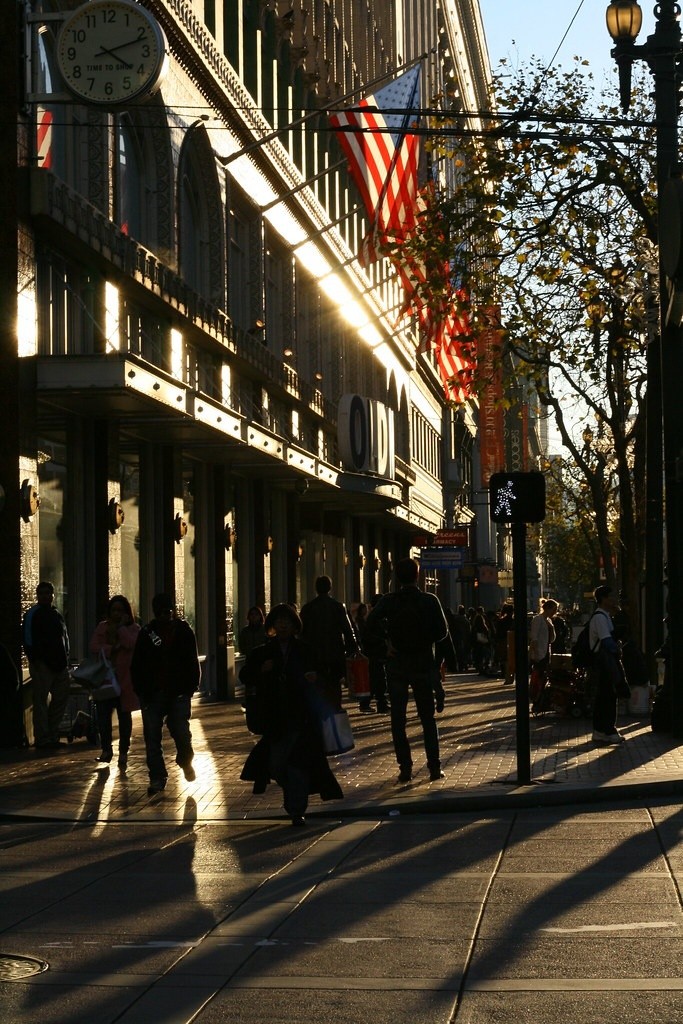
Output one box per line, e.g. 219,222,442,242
571,610,607,668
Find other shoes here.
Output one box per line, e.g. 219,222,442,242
430,773,444,781
118,754,128,765
148,785,161,796
435,691,445,713
377,706,391,714
183,762,193,780
293,815,306,826
359,704,376,713
96,756,111,763
399,773,412,781
37,741,67,752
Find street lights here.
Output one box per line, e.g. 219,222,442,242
604,0,682,743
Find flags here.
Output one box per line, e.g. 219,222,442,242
330,61,449,316
419,237,478,409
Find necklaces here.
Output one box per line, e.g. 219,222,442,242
278,636,293,680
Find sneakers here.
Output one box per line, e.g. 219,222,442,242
592,730,625,743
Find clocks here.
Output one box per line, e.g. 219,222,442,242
52,0,170,113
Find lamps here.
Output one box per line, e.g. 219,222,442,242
283,348,292,361
255,320,264,330
315,373,322,381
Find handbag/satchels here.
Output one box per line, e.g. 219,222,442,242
477,632,488,642
529,667,542,701
320,700,354,755
346,650,370,697
70,649,123,700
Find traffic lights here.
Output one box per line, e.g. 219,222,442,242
490,469,549,527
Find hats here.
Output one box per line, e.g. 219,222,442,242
594,584,616,601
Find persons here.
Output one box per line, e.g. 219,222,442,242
444,602,513,677
22,581,71,750
434,632,459,712
528,598,568,712
129,594,201,791
361,558,448,781
589,585,625,743
238,607,267,708
299,576,361,709
354,602,368,628
240,603,343,824
88,596,142,764
359,593,391,714
